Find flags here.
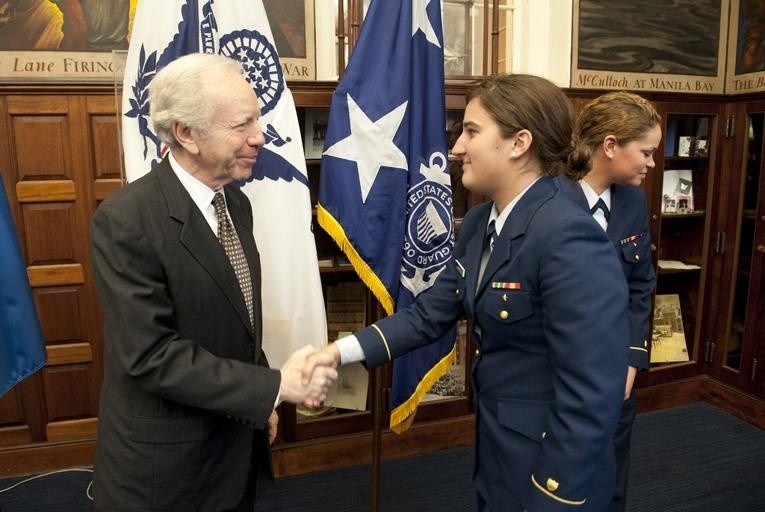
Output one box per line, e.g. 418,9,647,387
0,178,48,399
122,0,328,369
313,0,456,437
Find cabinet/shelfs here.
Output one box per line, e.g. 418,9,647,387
715,99,764,402
272,103,723,448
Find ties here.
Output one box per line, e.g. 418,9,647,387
209,191,257,329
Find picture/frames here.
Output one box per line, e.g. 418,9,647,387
441,0,474,80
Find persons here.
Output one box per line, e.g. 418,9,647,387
83,54,340,511
558,92,666,512
299,70,628,511
